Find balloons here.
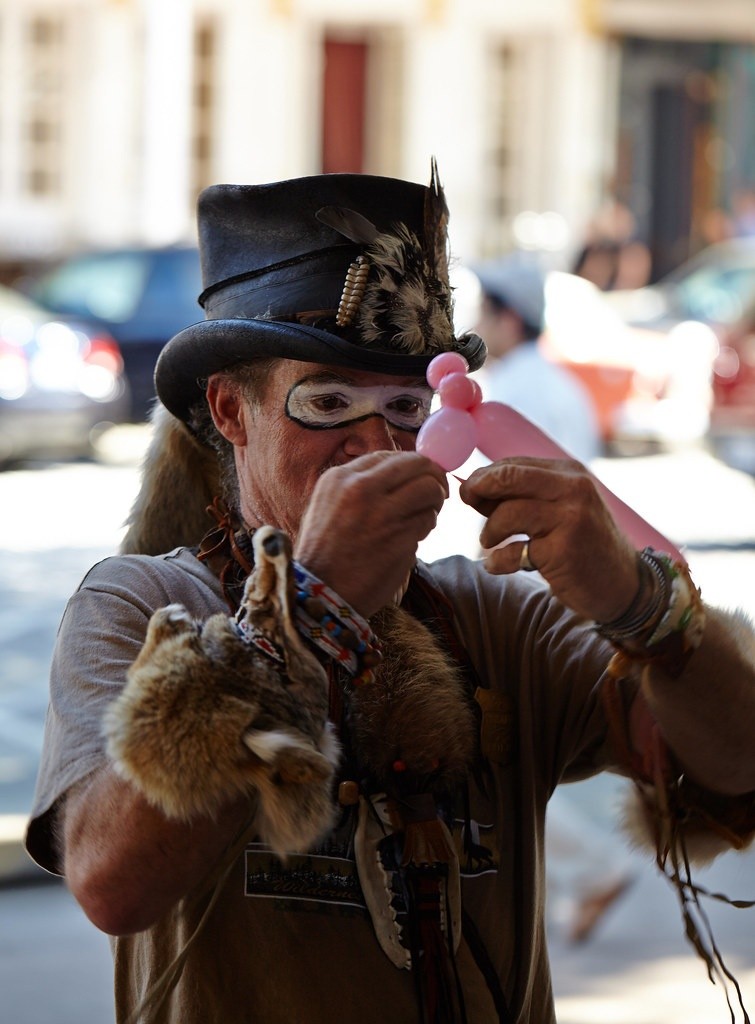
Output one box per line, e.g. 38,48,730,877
413,350,692,578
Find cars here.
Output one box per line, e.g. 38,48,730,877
25,245,208,426
447,270,685,456
0,284,133,469
606,235,755,433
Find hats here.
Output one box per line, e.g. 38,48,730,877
154,156,488,415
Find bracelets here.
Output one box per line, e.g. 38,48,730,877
231,554,388,694
594,545,673,639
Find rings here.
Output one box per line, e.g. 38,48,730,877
518,540,537,572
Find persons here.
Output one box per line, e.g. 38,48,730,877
21,165,755,1024
466,241,650,460
